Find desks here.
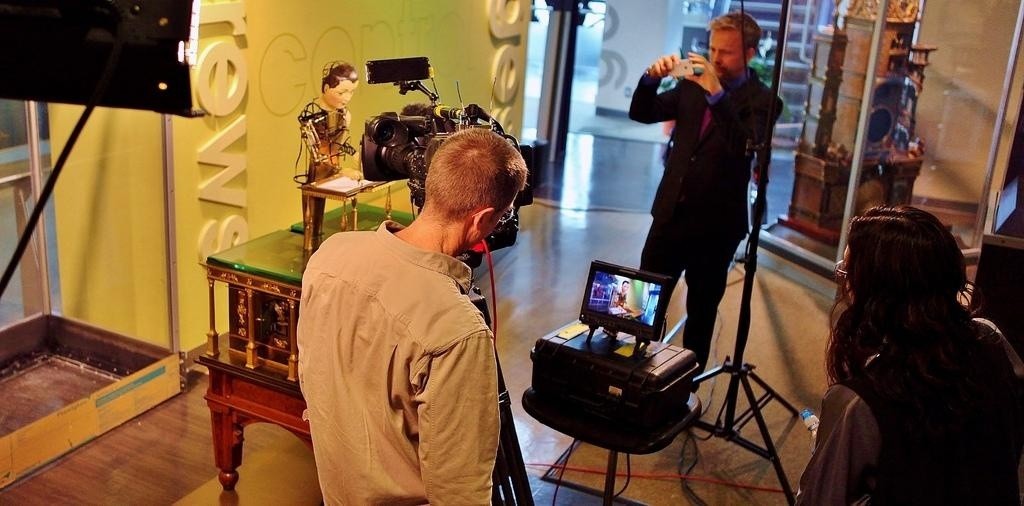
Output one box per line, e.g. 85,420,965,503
192,200,421,491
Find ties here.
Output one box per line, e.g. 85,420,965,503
696,105,714,146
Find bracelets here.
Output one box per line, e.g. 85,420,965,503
642,67,651,78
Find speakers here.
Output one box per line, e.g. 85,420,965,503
832,72,904,153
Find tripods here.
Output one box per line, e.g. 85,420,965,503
692,0,799,505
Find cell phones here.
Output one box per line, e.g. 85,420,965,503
668,59,695,78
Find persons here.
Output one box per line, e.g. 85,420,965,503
301,60,359,271
629,10,784,393
296,126,529,505
795,201,1024,505
614,280,629,308
